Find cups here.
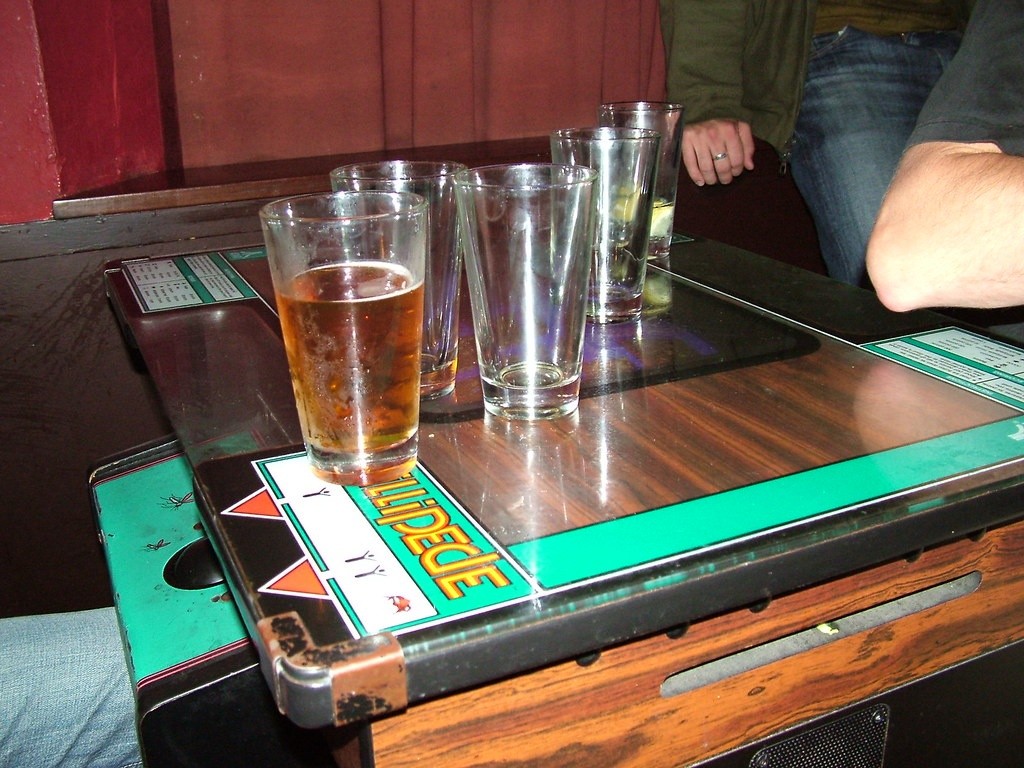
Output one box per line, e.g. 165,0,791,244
599,99,684,260
450,162,600,421
259,187,429,486
328,160,469,401
550,125,662,325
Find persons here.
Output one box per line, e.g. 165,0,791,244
657,0,974,286
0,607,145,768
866,0,1024,312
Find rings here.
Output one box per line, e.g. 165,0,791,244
713,153,727,161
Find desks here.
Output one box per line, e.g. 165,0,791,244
87,193,1024,768
